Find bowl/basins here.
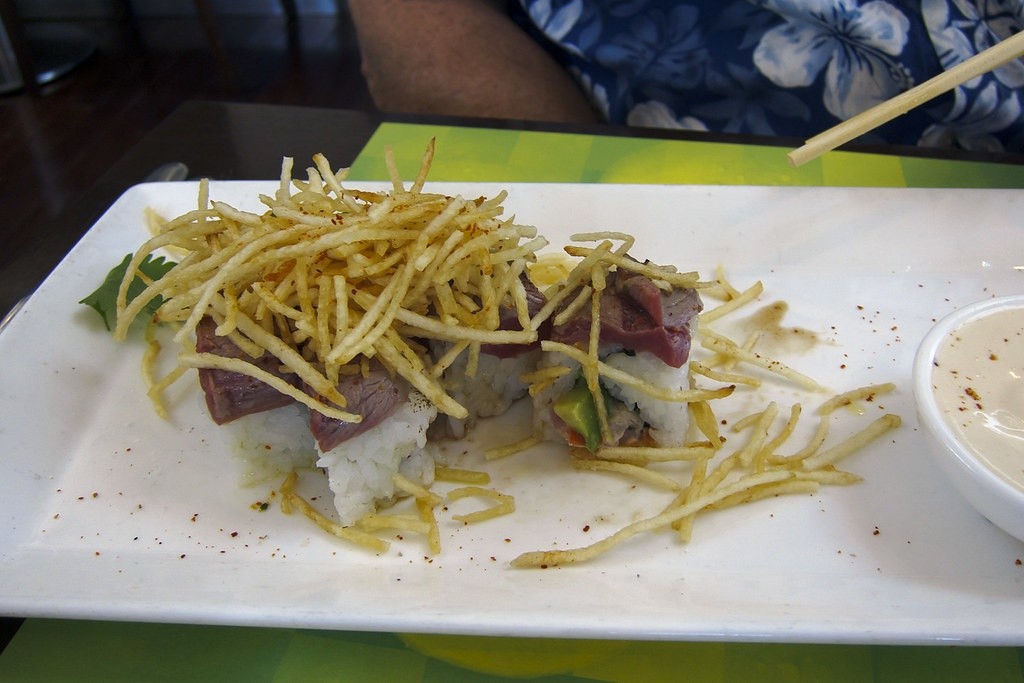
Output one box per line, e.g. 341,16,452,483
913,296,1023,546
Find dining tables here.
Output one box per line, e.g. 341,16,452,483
0,97,1024,679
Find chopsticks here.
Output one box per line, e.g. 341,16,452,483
787,29,1024,168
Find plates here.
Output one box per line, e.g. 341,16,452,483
0,181,1024,649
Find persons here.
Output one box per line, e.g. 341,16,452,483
348,0,1023,152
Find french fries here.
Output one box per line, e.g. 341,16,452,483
115,135,904,569
